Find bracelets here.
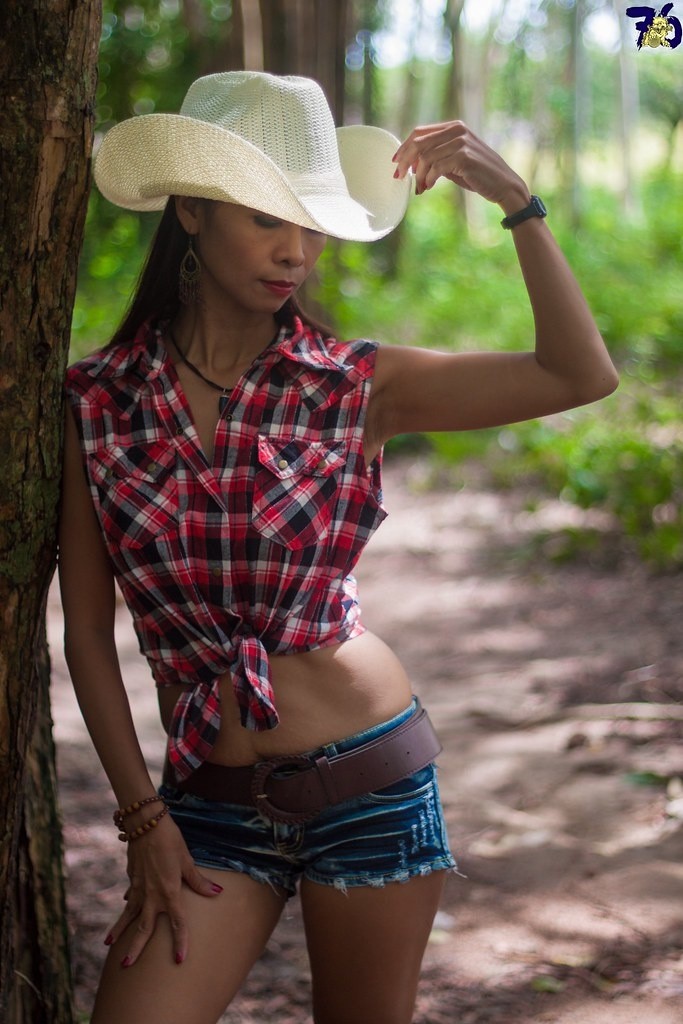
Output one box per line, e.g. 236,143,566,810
113,796,164,832
118,806,169,842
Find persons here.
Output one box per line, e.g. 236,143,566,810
61,69,621,1023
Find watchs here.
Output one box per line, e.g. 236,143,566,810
500,195,548,230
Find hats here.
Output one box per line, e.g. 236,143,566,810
92,70,413,244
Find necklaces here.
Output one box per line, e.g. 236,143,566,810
170,328,279,416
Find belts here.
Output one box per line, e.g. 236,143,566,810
162,695,444,826
